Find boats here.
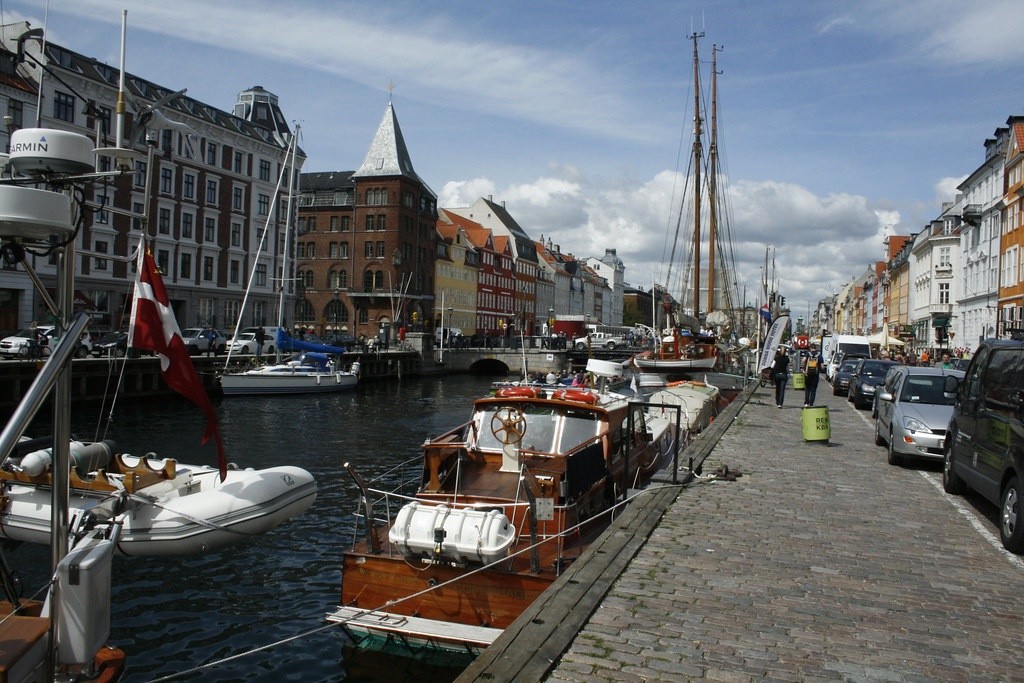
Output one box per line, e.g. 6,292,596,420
321,359,717,662
0,6,318,683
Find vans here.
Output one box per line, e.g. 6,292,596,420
941,338,1024,552
828,335,872,364
242,327,284,339
435,327,466,345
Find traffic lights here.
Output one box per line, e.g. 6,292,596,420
781,296,786,306
771,293,775,302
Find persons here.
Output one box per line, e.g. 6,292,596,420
771,346,791,408
871,347,969,371
628,327,715,347
28,320,44,359
251,327,265,357
803,343,824,406
551,331,578,350
523,369,625,388
207,328,217,357
359,326,406,353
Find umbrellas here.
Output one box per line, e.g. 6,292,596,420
867,321,904,352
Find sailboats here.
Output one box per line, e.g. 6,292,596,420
633,32,747,370
219,121,360,394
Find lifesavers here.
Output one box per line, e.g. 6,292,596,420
496,386,540,397
689,380,707,387
554,389,600,403
667,380,686,387
252,358,256,364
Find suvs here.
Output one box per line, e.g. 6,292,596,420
180,327,227,357
0,325,92,360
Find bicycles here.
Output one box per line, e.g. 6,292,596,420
18,337,52,360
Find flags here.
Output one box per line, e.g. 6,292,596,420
758,274,773,324
127,233,227,484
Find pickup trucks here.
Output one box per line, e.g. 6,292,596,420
575,333,627,350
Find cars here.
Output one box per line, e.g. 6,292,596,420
872,365,968,465
847,358,900,409
826,351,871,395
91,330,130,357
226,333,275,355
751,344,791,356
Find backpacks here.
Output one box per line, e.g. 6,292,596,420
805,351,821,373
